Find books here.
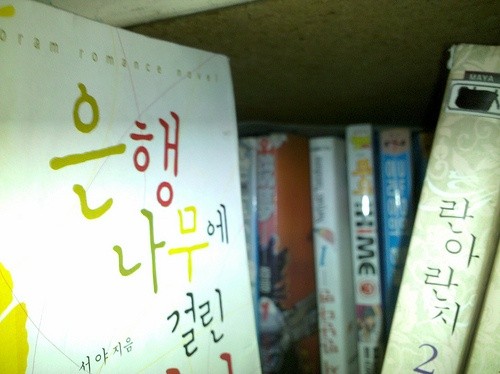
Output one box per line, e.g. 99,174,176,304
0,0,265,374
219,40,500,374
